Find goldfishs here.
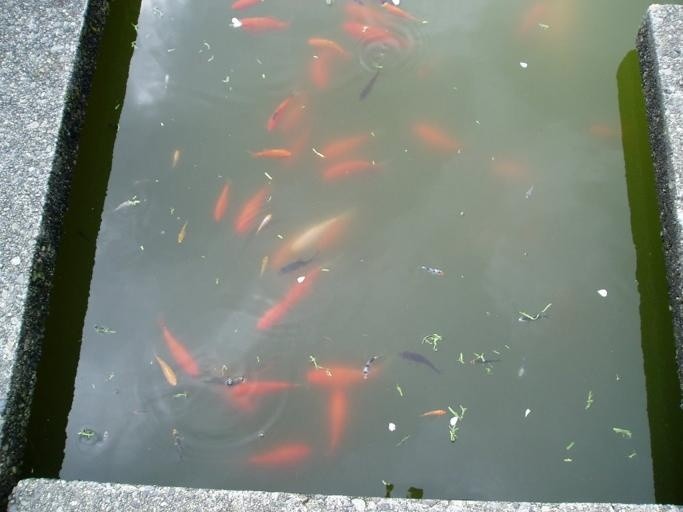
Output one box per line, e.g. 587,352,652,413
153,0,480,474
